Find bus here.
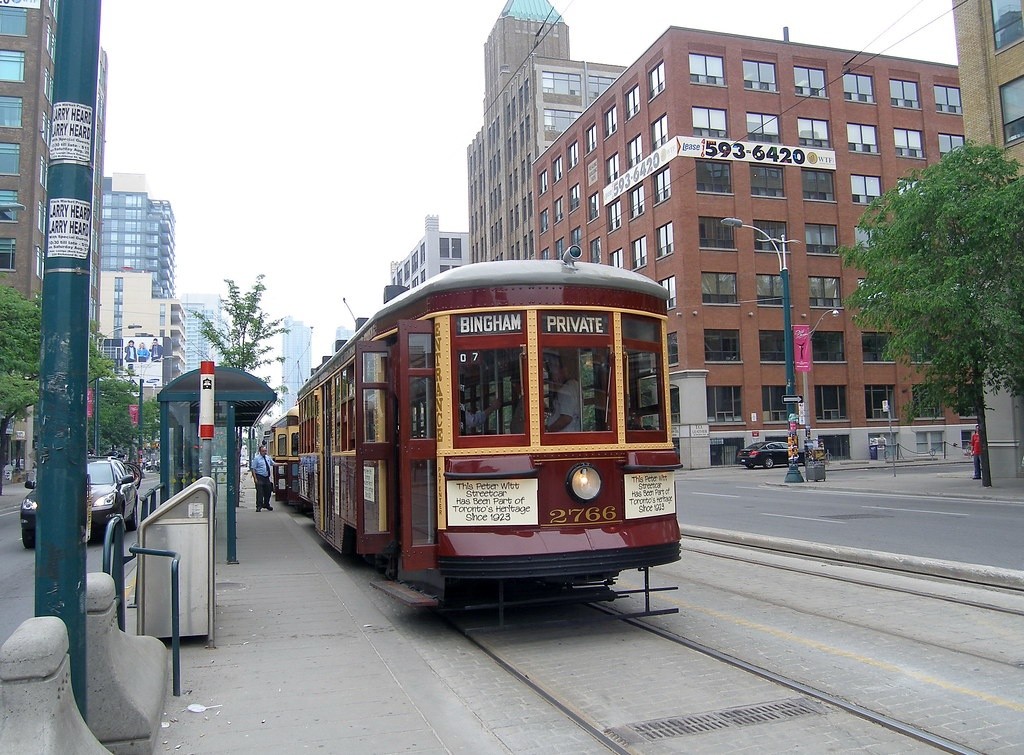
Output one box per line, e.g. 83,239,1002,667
295,243,685,638
263,401,299,511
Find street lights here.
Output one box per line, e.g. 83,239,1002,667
93,324,143,456
720,217,841,484
137,355,175,479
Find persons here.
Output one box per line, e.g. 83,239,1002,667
250,445,288,512
970,425,983,479
459,391,502,425
546,357,581,432
507,372,525,435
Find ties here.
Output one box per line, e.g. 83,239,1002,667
263,456,270,477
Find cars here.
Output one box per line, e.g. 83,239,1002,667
145,455,224,471
19,454,139,549
737,441,814,469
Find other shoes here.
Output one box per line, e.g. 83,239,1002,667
256,506,262,512
973,476,981,479
263,503,273,511
